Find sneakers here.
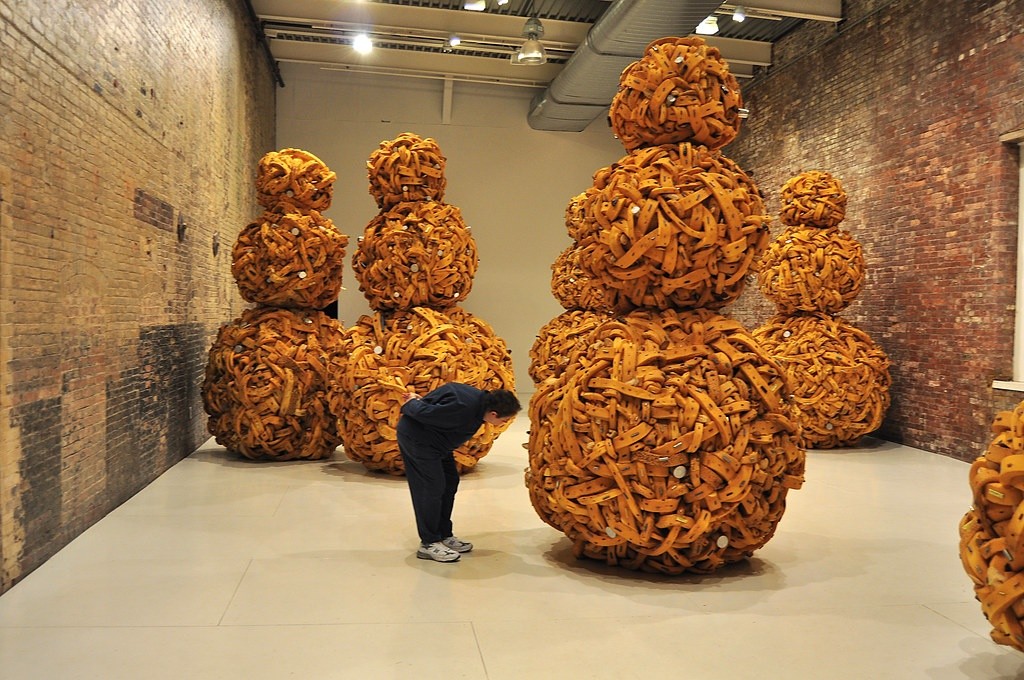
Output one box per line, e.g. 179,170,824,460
417,539,461,562
442,534,473,554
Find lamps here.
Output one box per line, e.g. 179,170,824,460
519,2,548,66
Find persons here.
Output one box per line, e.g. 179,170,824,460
396,383,522,562
